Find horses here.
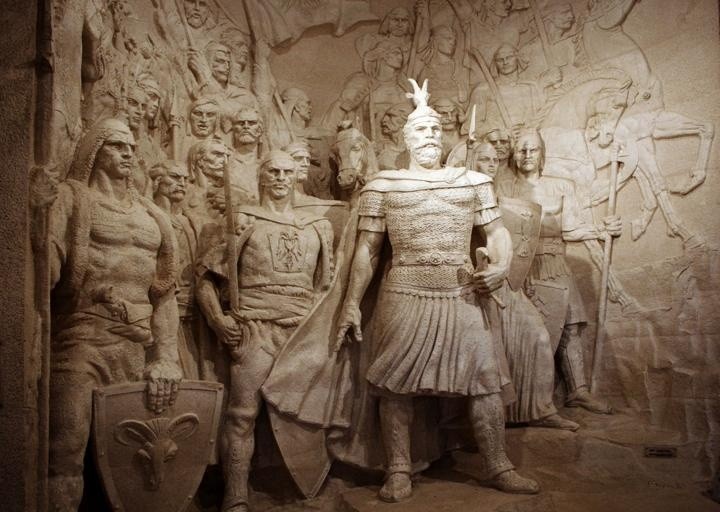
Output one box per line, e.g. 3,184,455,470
444,66,645,319
572,0,715,253
327,115,381,209
